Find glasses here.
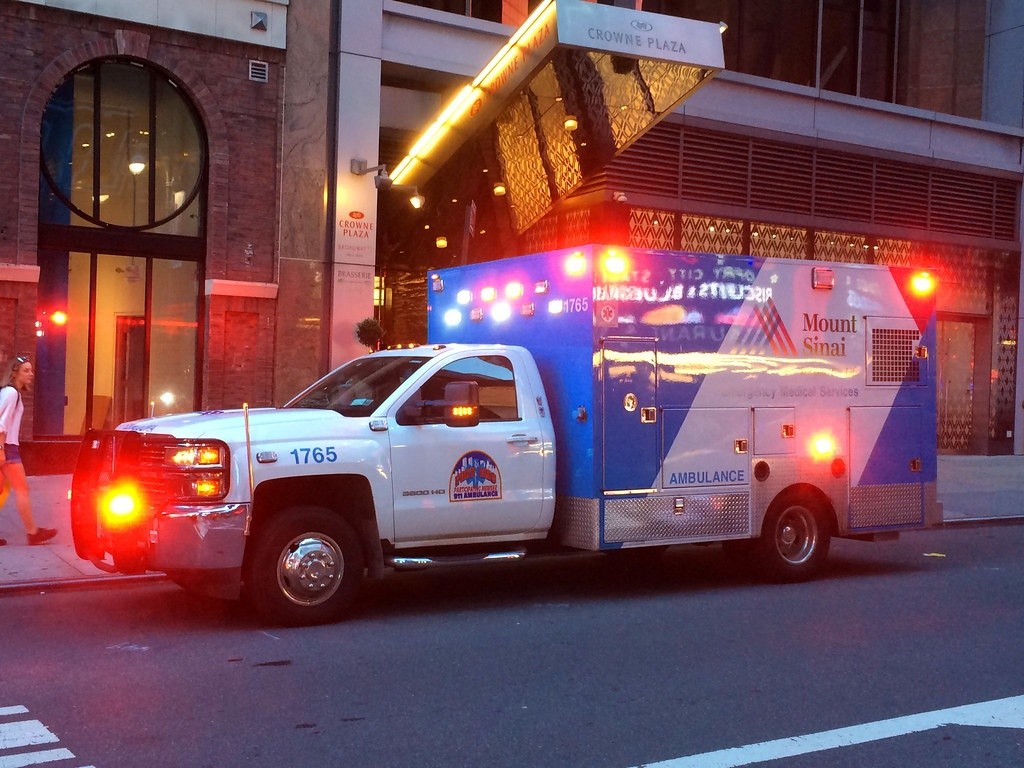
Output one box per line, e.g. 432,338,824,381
16,356,27,363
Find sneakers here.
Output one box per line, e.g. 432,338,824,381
27,528,58,545
0,539,7,545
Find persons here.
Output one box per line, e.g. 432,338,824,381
0,354,58,546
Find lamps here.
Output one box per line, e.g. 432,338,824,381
128,154,146,176
351,158,392,190
494,182,506,197
436,237,447,248
391,184,426,210
563,115,578,130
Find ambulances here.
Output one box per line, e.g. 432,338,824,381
70,243,940,622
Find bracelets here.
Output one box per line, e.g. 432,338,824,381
0,445,4,448
